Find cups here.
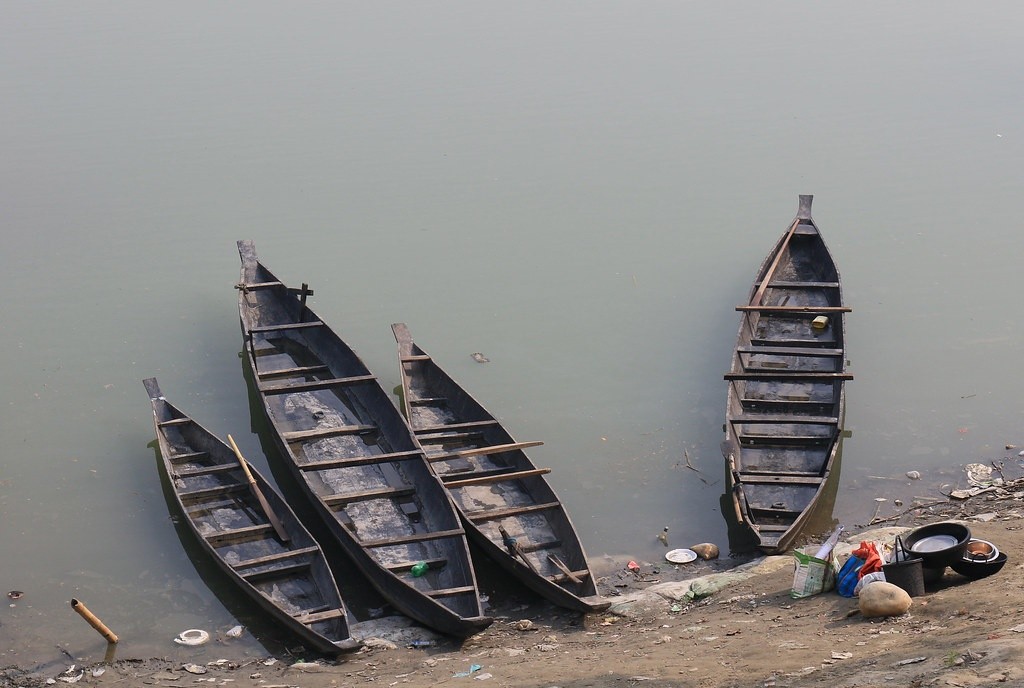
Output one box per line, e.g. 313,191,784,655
411,561,428,575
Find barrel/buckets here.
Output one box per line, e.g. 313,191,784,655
880,535,925,599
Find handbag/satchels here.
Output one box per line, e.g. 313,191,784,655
790,543,834,599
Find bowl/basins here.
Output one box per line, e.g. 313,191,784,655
966,541,994,559
901,522,972,559
963,539,999,562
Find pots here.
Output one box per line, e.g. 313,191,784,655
950,551,1007,577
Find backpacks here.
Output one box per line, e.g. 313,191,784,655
835,554,866,597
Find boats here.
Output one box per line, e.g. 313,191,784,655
234,235,494,641
721,189,855,559
137,376,366,654
385,317,612,616
